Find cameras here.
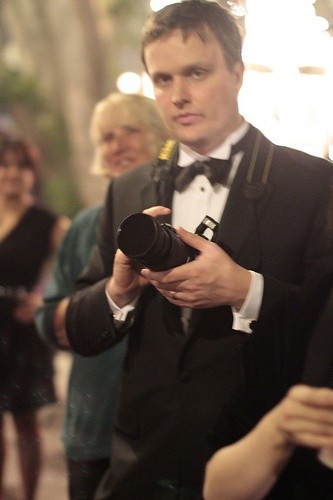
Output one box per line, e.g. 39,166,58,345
116,213,200,272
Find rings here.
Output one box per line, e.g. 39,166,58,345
168,290,178,299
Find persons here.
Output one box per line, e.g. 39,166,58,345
179,268,332,500
65,1,333,500
32,92,177,500
1,138,73,500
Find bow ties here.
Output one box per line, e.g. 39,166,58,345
174,158,233,194
